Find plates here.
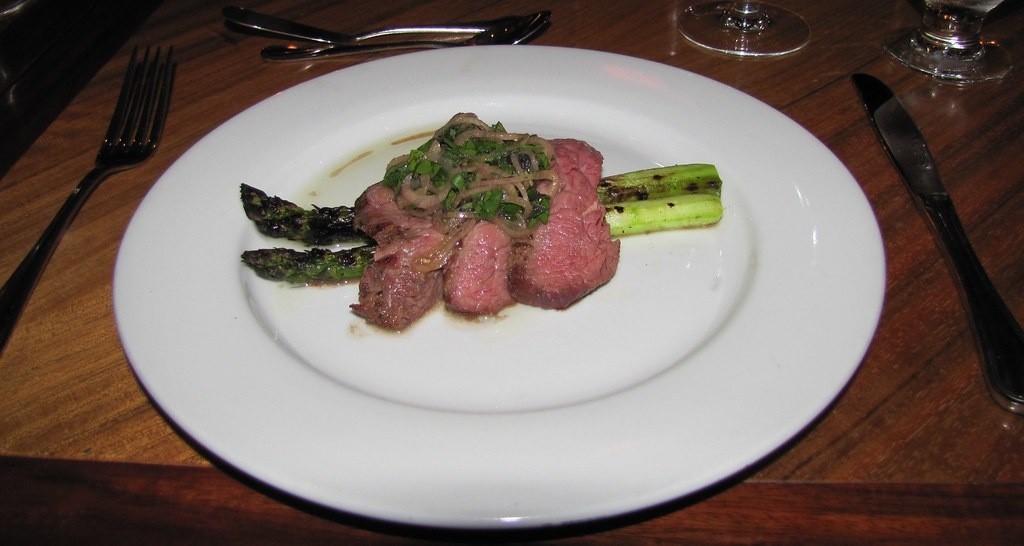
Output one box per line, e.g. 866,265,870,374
115,48,887,527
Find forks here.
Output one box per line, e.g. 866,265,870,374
0,49,176,355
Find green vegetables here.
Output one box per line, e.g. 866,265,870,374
384,124,549,228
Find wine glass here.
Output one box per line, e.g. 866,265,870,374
884,0,1013,84
676,0,811,56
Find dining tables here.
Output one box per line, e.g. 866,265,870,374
0,6,1024,546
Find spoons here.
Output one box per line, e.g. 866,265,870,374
260,11,552,62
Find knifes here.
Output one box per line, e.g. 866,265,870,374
851,70,1024,413
